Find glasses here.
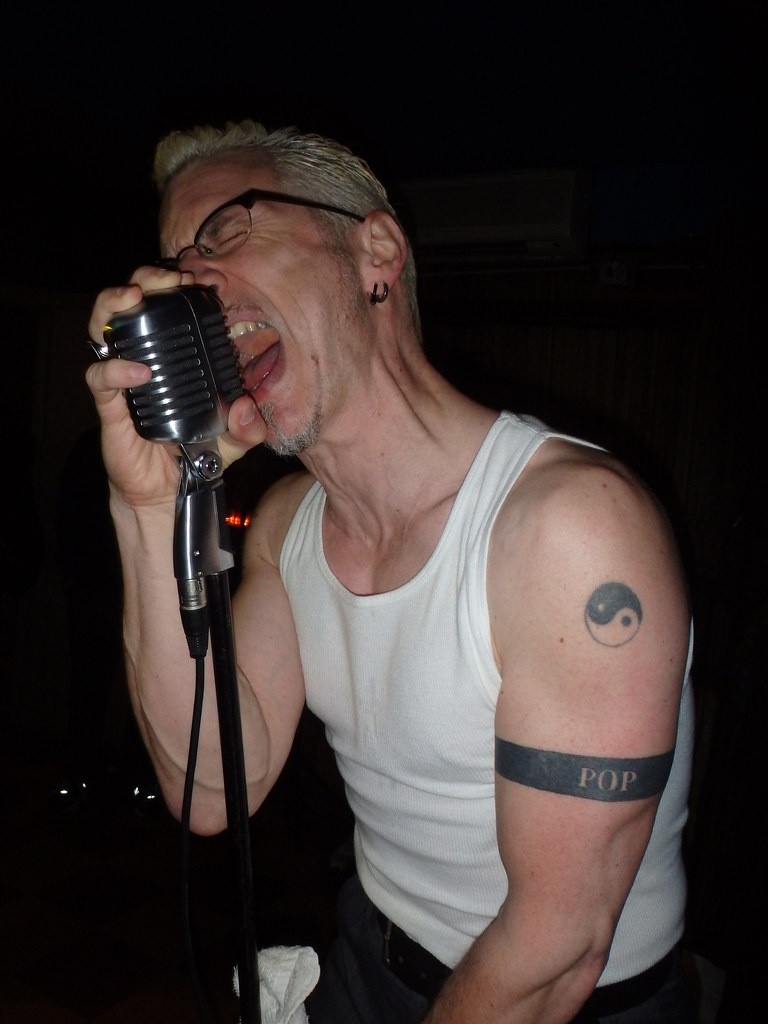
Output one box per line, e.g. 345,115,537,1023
155,187,365,273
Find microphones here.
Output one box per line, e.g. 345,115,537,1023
102,284,247,658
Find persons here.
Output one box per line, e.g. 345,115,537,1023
85,116,722,1024
